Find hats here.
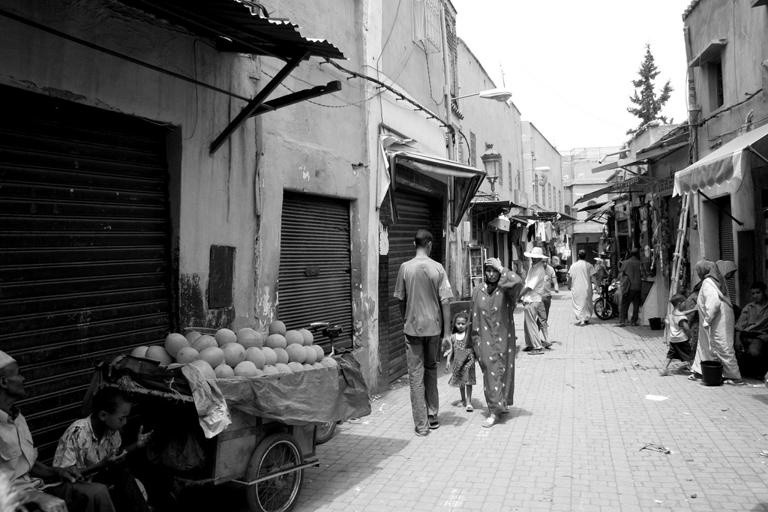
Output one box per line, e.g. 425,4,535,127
523,247,549,259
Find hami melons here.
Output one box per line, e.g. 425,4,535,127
130,320,338,380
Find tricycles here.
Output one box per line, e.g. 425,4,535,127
96,320,354,512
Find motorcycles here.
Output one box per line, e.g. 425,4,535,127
592,283,618,320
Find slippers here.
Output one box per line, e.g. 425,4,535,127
723,379,744,385
659,368,703,381
522,318,638,355
414,400,510,437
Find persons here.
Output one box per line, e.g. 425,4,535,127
660,260,768,388
516,246,642,355
52,386,180,512
0,351,116,512
467,257,522,428
393,228,455,436
445,311,476,412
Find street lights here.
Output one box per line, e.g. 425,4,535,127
511,165,550,207
443,86,515,296
477,140,508,260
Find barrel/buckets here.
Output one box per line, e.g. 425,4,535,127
700,360,723,385
649,317,661,330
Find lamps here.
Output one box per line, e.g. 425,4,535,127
481,143,503,197
453,87,513,102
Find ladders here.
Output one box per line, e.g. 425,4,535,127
468,245,486,298
664,194,692,345
